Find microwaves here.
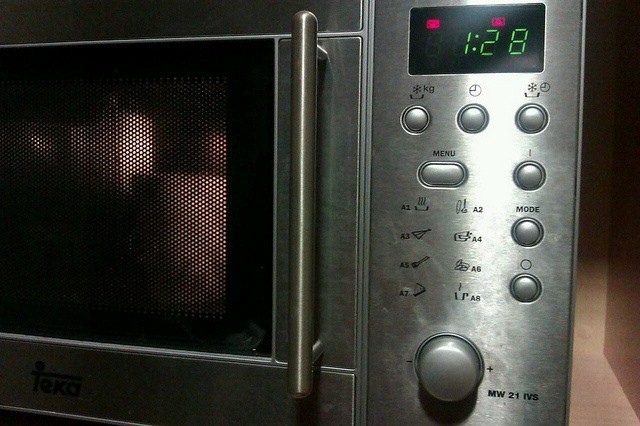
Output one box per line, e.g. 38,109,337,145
0,0,587,426
